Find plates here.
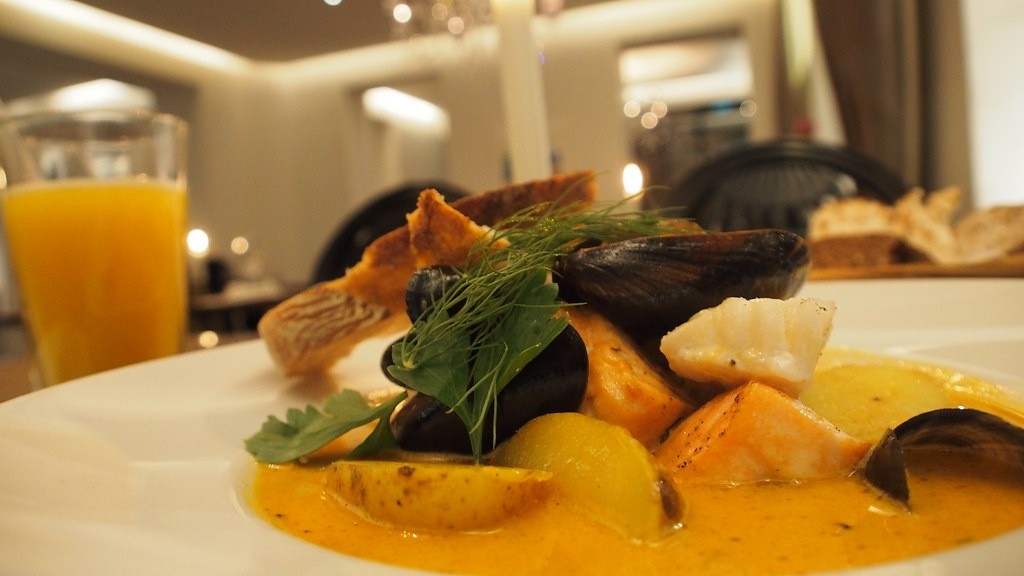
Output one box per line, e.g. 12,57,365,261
0,277,1024,576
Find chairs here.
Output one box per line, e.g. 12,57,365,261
650,138,918,243
307,180,474,289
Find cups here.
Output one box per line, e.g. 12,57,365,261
0,113,189,390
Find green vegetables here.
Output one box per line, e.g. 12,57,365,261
242,173,721,466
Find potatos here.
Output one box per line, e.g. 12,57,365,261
325,298,873,544
